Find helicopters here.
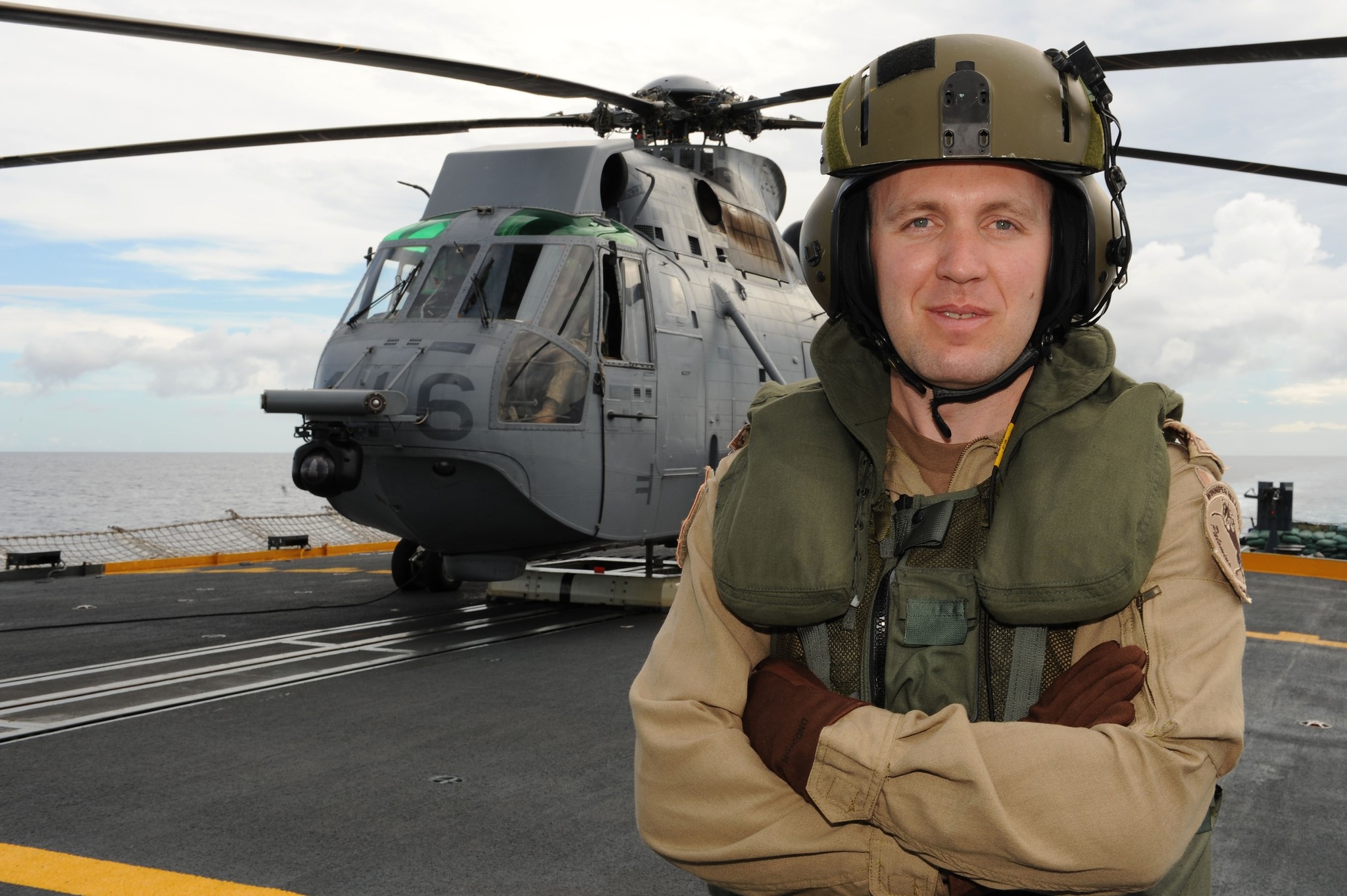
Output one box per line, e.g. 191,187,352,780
1,4,1347,600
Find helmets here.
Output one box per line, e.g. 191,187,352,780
552,257,580,274
811,30,1127,327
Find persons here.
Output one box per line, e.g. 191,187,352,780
630,64,1250,895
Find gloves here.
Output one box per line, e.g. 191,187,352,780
1015,638,1151,729
743,652,862,796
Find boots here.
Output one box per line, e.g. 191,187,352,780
533,401,556,424
